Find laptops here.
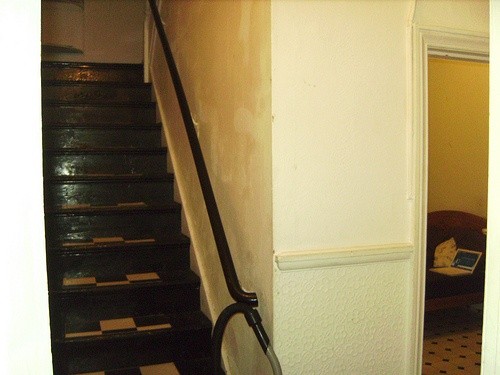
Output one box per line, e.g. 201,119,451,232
429,247,483,277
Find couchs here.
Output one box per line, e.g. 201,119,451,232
425,210,487,312
